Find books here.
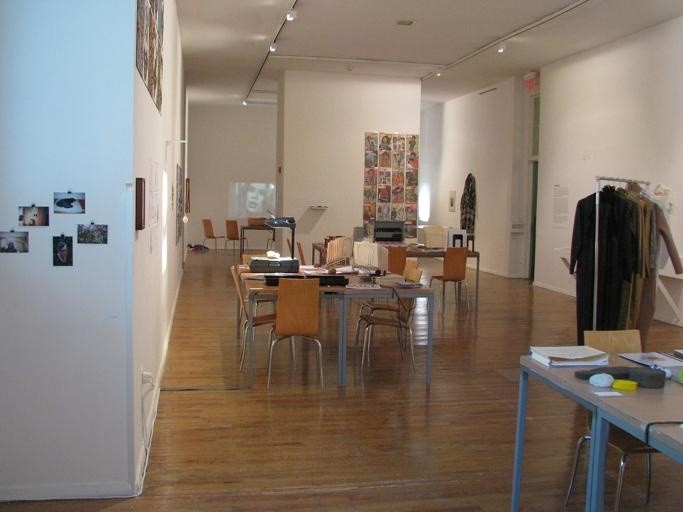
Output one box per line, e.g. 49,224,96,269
299,263,422,289
549,357,611,366
616,351,683,368
672,348,683,357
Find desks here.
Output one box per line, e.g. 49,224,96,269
508,353,682,511
404,245,480,311
239,224,275,257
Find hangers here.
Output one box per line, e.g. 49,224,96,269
577,177,664,210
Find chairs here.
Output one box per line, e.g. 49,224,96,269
230,246,470,393
201,218,247,255
564,330,662,511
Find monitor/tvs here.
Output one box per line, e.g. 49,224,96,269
372,220,404,243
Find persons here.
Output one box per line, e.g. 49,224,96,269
6,242,17,252
56,242,68,266
236,183,274,216
364,133,418,238
